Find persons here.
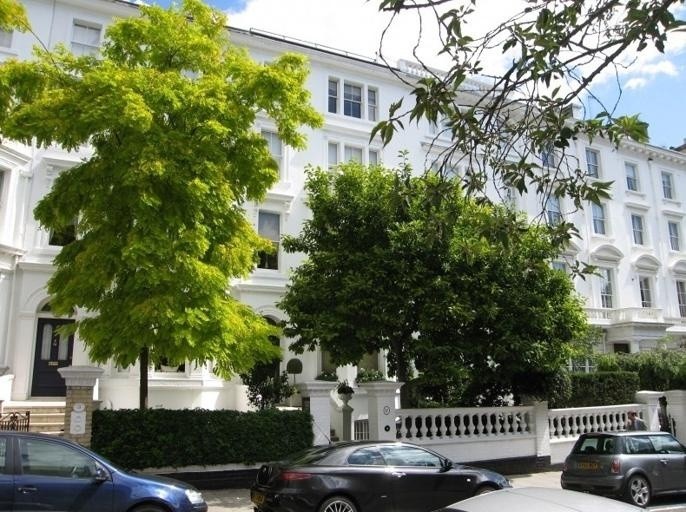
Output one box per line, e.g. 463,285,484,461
624,408,649,432
657,395,678,437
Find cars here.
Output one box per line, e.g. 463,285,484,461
431,486,649,512
0,430,208,512
249,439,516,512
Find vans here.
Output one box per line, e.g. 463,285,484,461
555,430,685,509
